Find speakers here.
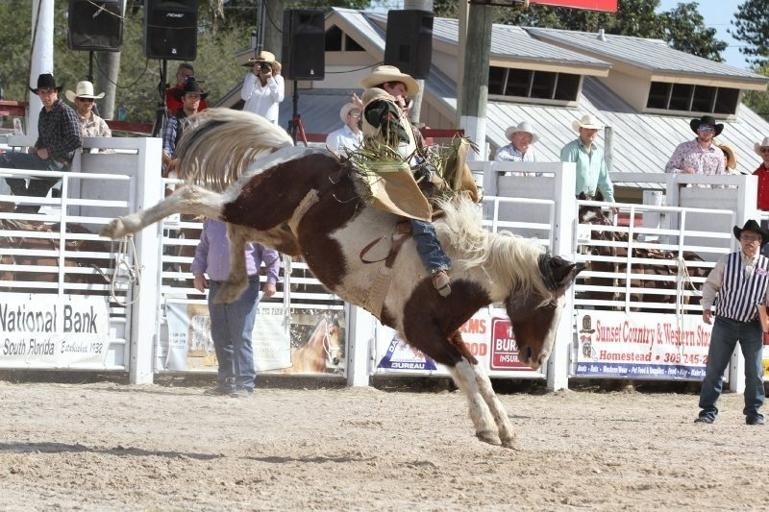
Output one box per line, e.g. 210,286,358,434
144,0,199,63
279,9,328,82
382,9,433,78
67,0,123,50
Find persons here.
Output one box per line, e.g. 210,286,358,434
749,137,769,255
0,75,82,217
719,143,740,189
666,115,726,190
190,216,281,399
360,65,453,297
327,103,366,164
241,52,285,126
65,81,112,152
560,114,619,253
494,121,546,177
162,79,210,177
166,64,209,113
694,220,769,425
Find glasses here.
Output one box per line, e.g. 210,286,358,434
76,96,94,102
760,147,769,153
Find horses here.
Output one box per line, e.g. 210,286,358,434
107,108,589,450
269,310,343,374
577,191,713,394
0,219,128,295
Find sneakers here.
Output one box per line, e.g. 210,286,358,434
204,384,236,397
231,385,254,398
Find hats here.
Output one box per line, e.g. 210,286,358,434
65,80,106,103
361,63,419,98
175,78,211,101
241,51,281,73
718,144,736,169
734,219,769,244
572,114,603,132
505,121,540,144
340,102,362,124
690,115,724,137
754,136,769,157
29,73,65,95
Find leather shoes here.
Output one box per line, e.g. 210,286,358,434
751,419,764,425
694,416,713,424
432,269,451,298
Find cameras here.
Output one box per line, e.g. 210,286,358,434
257,62,275,79
181,71,200,87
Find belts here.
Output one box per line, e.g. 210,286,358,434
715,314,758,327
